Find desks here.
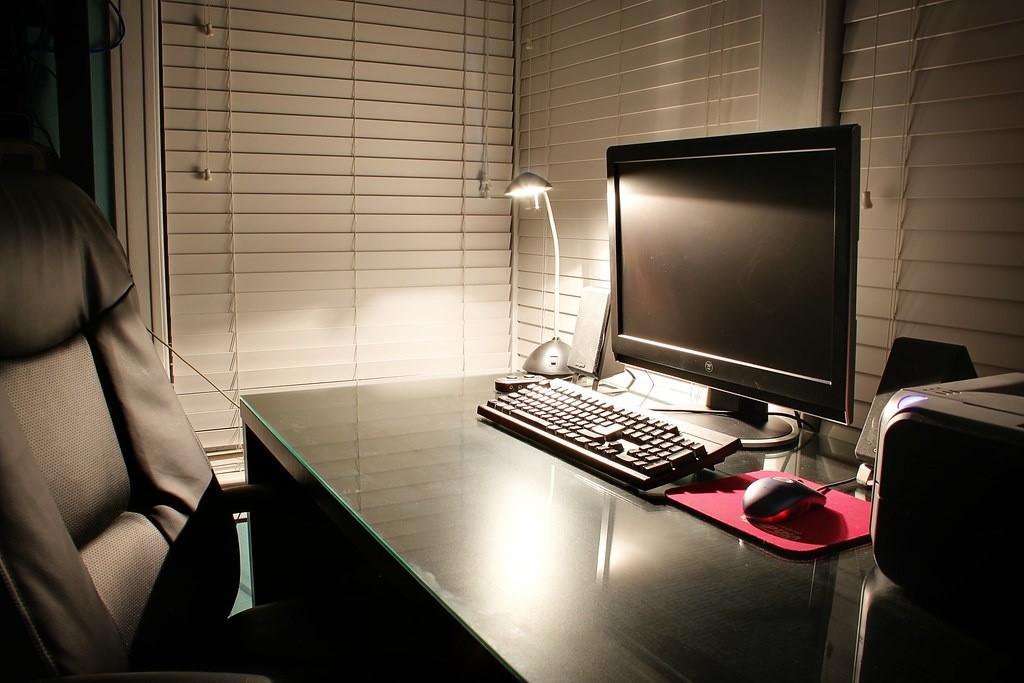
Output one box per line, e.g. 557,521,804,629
239,373,1024,683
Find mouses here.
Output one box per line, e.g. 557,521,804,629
741,477,827,524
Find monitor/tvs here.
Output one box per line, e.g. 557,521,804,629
607,123,861,449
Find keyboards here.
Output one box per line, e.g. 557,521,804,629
477,379,743,492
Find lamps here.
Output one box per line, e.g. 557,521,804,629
503,172,585,381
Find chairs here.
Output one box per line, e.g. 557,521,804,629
0,168,458,683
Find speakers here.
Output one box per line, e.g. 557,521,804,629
856,336,979,487
567,288,628,396
